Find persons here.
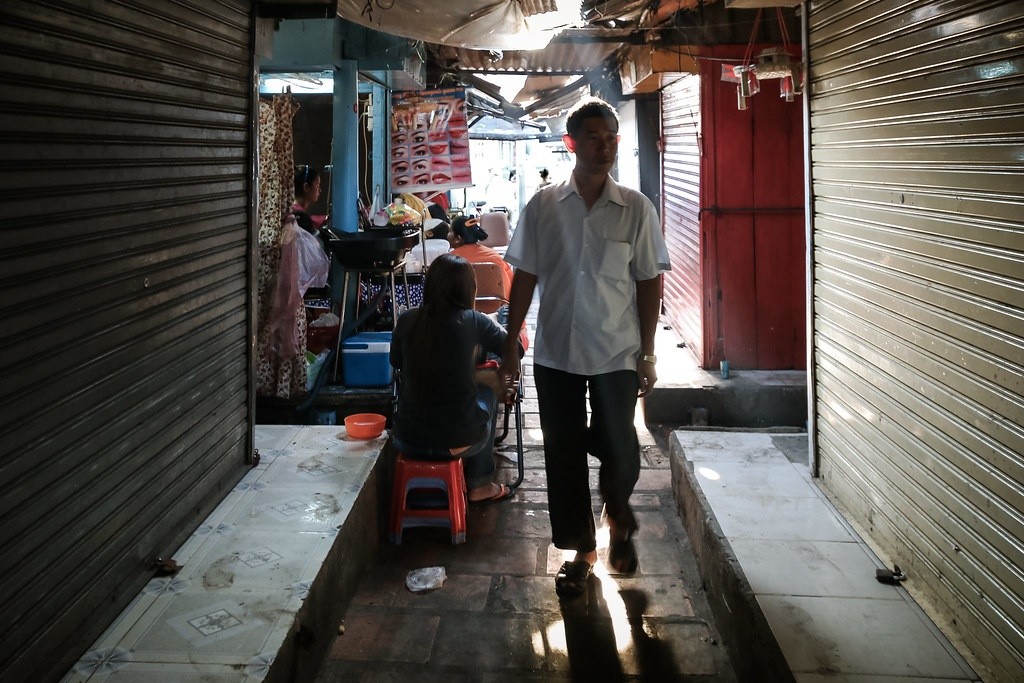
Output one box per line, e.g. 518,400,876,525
390,254,525,502
497,98,672,596
400,203,517,315
287,164,330,234
391,97,472,189
483,169,556,203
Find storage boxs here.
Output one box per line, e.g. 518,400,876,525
340,332,393,388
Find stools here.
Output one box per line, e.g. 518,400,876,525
386,452,469,546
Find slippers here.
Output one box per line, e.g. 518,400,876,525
554,558,595,597
609,513,639,573
470,483,517,506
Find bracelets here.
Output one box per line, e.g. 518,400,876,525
639,354,660,365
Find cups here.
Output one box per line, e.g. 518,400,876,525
719,360,729,379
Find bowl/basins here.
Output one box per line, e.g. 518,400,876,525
344,413,387,439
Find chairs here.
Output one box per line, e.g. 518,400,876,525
477,357,523,491
469,262,506,314
476,213,510,248
